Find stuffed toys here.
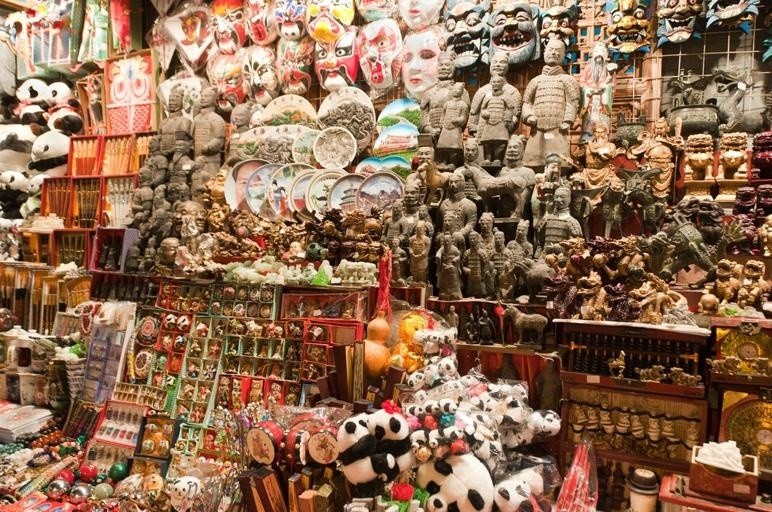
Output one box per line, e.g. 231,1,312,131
0,123,45,179
46,80,84,134
443,375,527,393
494,477,552,512
511,468,552,512
367,409,414,482
17,80,48,125
337,413,396,487
22,174,50,216
0,171,29,219
27,129,76,185
442,327,459,357
407,371,430,391
0,219,23,261
416,453,494,512
392,311,429,373
437,356,458,380
424,364,442,386
404,394,561,473
423,332,441,358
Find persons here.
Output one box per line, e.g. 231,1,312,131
507,219,533,260
484,261,499,300
407,135,435,191
599,461,613,494
157,88,192,161
145,235,156,272
401,178,432,222
531,153,572,229
381,202,409,247
0,308,16,330
488,231,513,271
227,103,252,154
479,211,494,246
434,82,469,174
631,116,685,213
571,121,626,216
610,462,626,502
171,183,190,211
537,183,583,266
152,184,172,210
409,205,434,238
208,202,225,231
497,134,536,214
435,232,463,300
139,196,173,238
167,130,196,185
377,243,393,320
189,85,225,177
453,138,482,201
407,220,431,287
235,209,254,230
461,230,490,300
481,75,517,165
175,201,207,233
446,305,459,326
390,237,408,286
522,39,580,166
126,237,141,274
233,161,270,211
579,41,618,141
126,159,154,228
191,156,211,200
158,237,179,266
145,135,168,186
440,173,477,250
495,262,516,302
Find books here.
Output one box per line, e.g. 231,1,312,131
0,399,58,445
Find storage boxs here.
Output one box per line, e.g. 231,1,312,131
690,444,759,504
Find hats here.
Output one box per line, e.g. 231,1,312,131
175,131,193,141
